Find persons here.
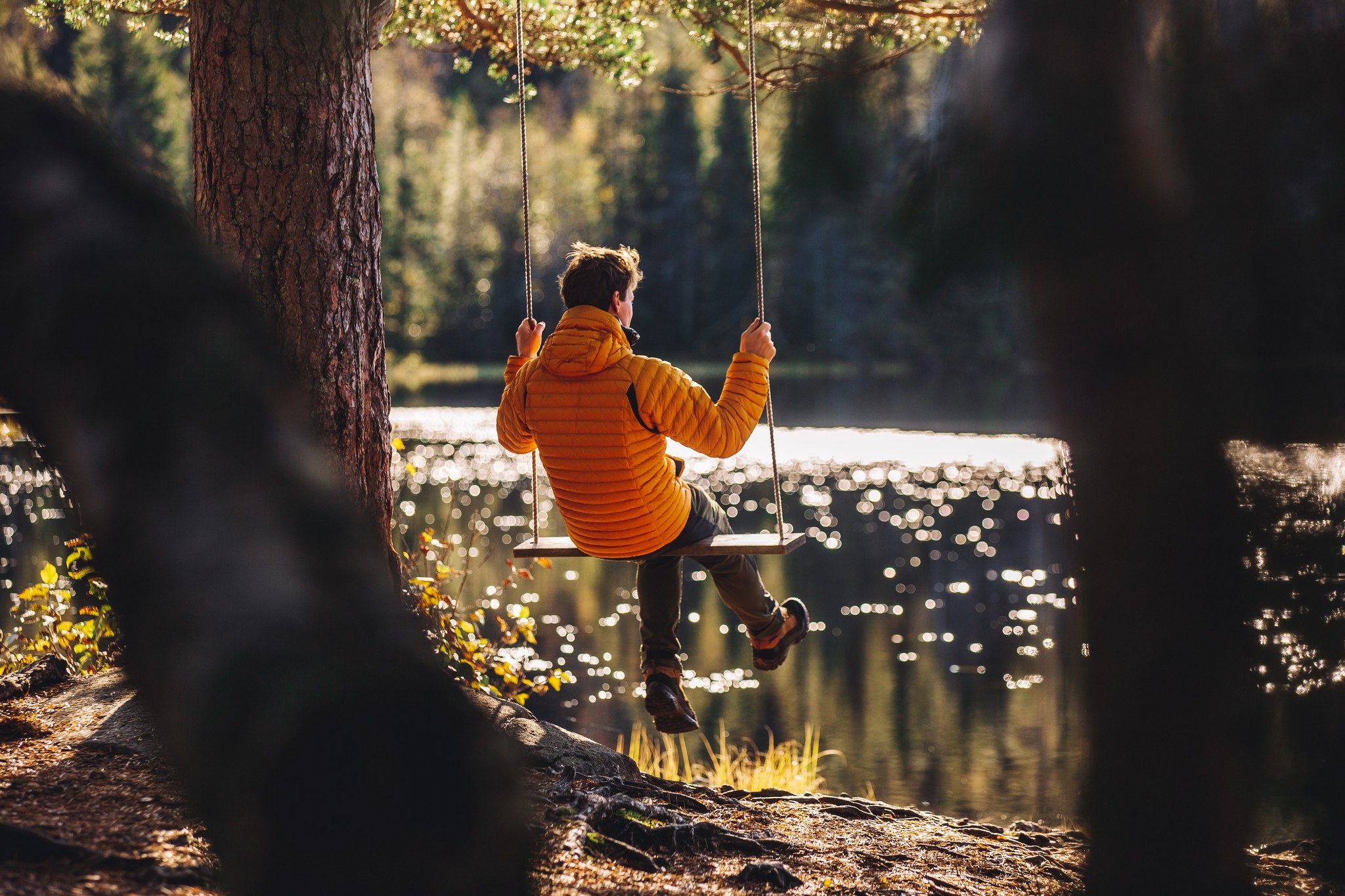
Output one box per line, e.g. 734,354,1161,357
496,240,809,735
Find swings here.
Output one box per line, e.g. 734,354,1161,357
508,2,813,561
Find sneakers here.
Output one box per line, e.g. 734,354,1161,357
643,667,699,734
750,597,810,671
